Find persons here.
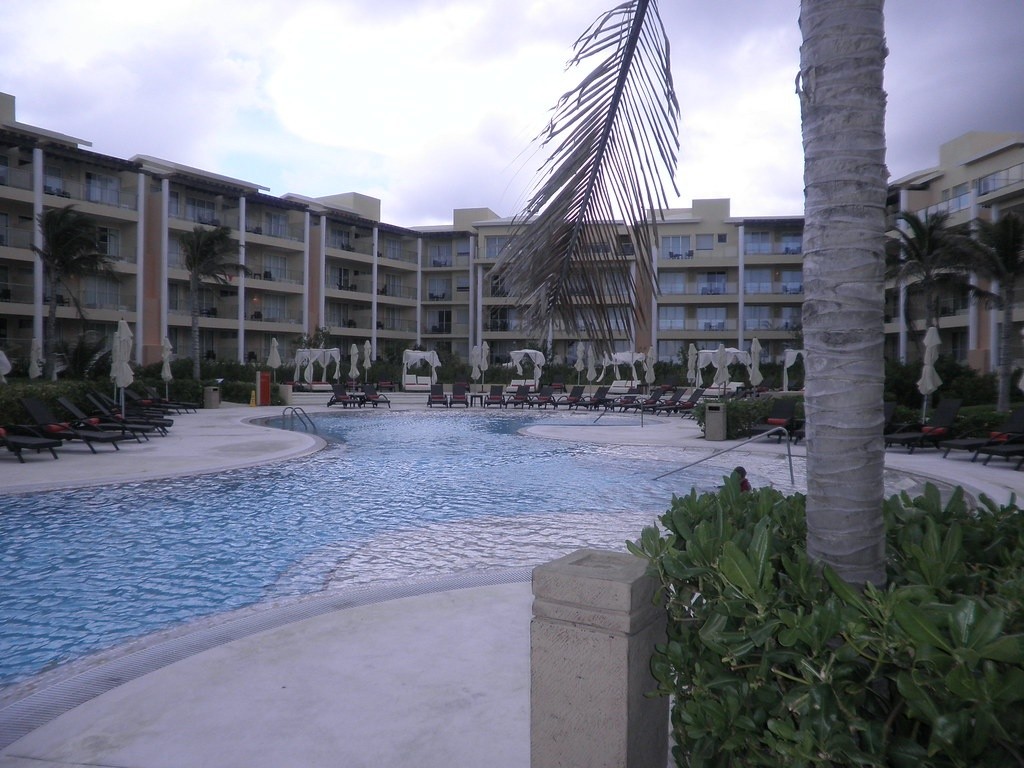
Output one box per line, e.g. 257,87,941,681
110,320,134,420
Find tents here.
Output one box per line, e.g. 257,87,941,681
696,347,752,389
595,351,646,389
510,349,546,389
294,347,341,384
782,348,806,392
402,349,441,392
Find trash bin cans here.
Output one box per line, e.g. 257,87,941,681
704,401,728,441
203,385,221,410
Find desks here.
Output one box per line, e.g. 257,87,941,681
470,395,483,407
349,395,365,408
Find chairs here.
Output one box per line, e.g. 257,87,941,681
327,380,396,409
736,386,805,445
427,382,705,420
0,385,200,463
884,399,1024,471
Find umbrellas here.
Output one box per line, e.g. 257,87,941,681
714,344,731,400
687,343,698,396
916,327,943,424
574,342,585,385
645,345,656,399
267,338,281,383
29,338,43,381
363,340,372,383
587,345,597,409
348,344,360,394
751,336,763,398
470,340,490,384
160,336,173,402
